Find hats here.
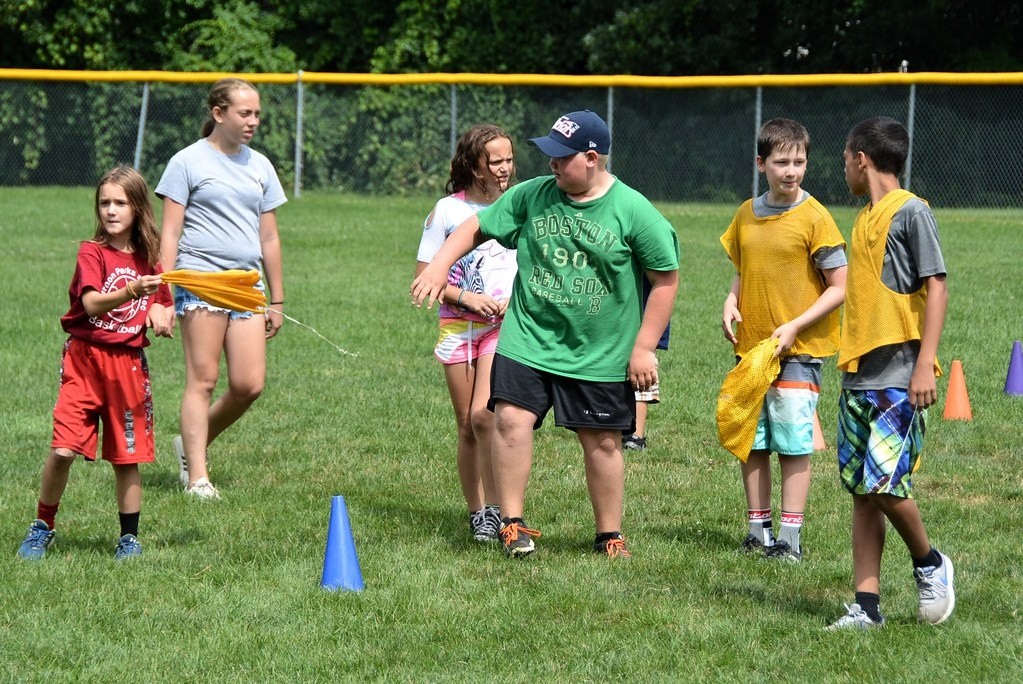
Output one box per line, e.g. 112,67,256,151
527,108,610,157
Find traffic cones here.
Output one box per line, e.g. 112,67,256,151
942,359,973,422
1004,341,1023,398
318,496,365,592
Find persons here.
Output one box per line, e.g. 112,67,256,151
152,79,289,502
18,169,176,563
415,124,520,543
408,111,680,562
715,118,847,567
828,118,955,632
624,271,671,452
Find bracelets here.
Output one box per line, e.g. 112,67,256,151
271,302,284,305
125,282,140,300
457,290,466,306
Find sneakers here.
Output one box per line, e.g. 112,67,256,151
184,476,223,499
761,540,803,562
743,533,763,550
593,531,632,560
17,519,57,560
623,434,647,451
497,516,541,558
469,505,501,541
818,601,885,631
913,549,955,624
114,534,141,560
172,436,189,484
